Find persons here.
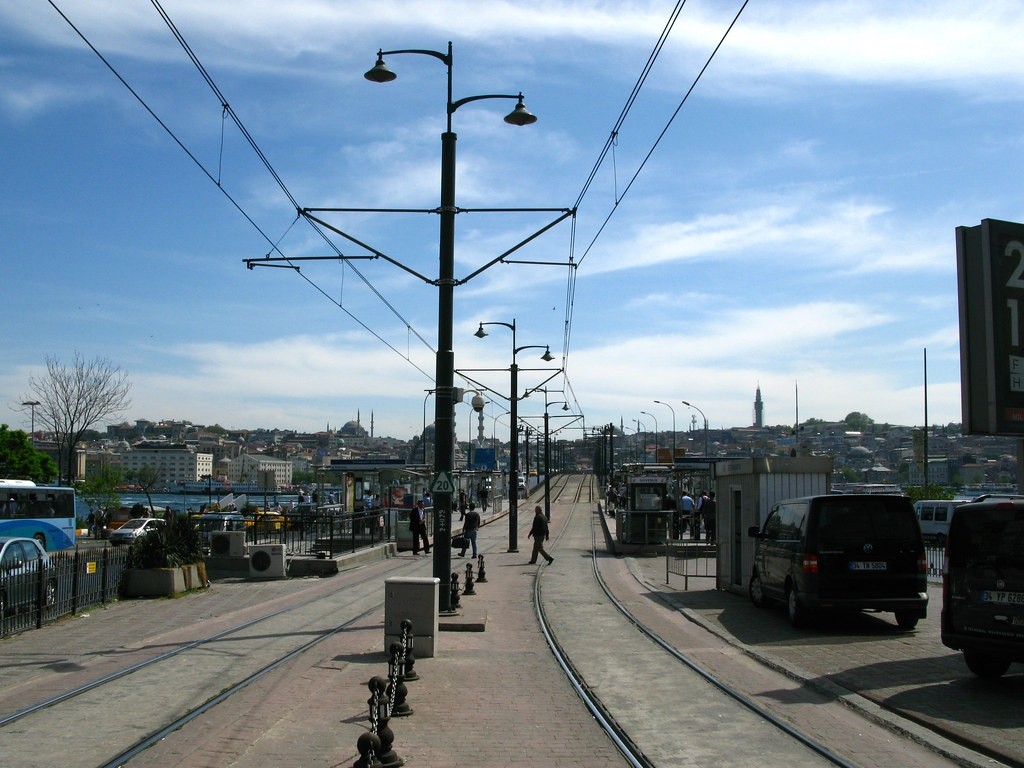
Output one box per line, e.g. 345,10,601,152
458,503,480,559
298,489,335,503
409,500,432,555
606,488,626,518
365,490,380,508
528,506,554,566
679,491,717,545
479,486,488,513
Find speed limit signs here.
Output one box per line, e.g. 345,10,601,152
431,471,456,493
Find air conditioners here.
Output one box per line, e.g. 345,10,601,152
211,530,245,559
249,545,287,577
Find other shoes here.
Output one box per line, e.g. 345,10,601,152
413,553,420,555
472,555,477,558
529,560,536,564
425,551,431,554
547,557,554,565
457,553,464,557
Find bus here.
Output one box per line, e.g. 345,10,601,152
0,478,79,553
103,504,168,538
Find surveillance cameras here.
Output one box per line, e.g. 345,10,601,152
472,396,485,413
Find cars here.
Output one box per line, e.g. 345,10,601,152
107,517,168,547
518,476,526,489
0,536,58,620
522,466,558,478
190,502,342,544
485,479,493,492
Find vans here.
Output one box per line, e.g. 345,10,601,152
939,492,1024,678
912,497,972,547
746,492,930,632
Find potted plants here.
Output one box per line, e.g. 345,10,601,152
124,511,208,595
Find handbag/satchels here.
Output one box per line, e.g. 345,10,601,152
452,534,470,548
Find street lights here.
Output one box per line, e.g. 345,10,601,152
21,400,41,448
492,411,511,463
641,411,658,463
470,318,556,554
201,474,212,510
682,401,708,458
631,419,647,462
523,386,572,524
360,38,538,614
625,426,638,463
467,401,491,471
653,401,676,457
